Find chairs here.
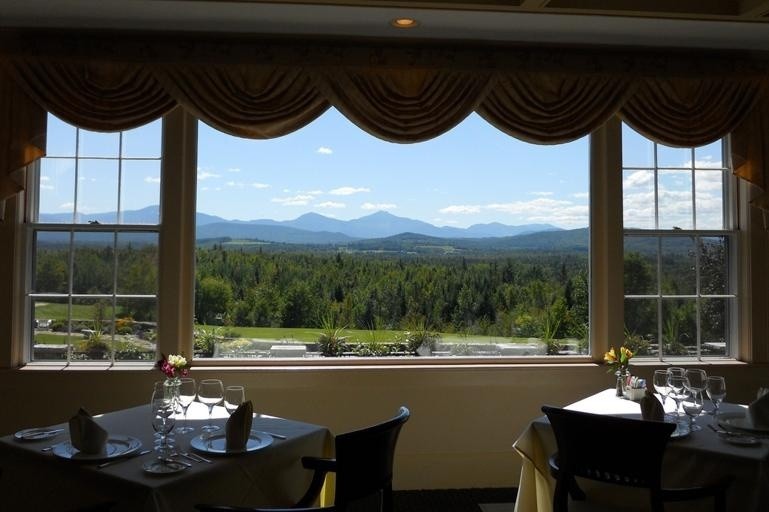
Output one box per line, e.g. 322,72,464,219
540,405,736,511
192,404,412,511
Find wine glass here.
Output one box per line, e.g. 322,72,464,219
652,366,727,438
150,378,245,463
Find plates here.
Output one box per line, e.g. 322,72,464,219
142,457,188,474
51,432,142,460
189,428,273,453
14,427,60,439
712,410,769,444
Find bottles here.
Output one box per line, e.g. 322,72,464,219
616,376,623,396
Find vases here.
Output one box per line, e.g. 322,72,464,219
162,378,182,398
616,375,626,396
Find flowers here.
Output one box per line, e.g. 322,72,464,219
154,352,192,397
602,344,634,393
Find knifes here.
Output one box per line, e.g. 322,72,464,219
155,447,213,467
98,450,152,468
